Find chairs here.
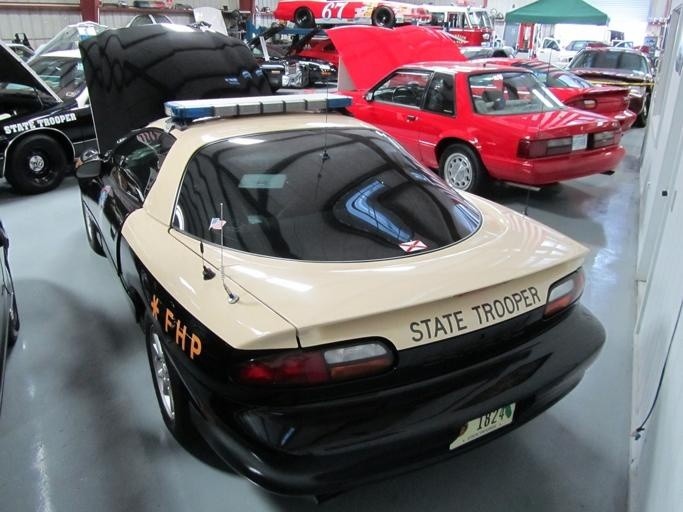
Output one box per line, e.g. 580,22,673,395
430,78,503,113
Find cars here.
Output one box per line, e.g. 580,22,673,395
63,23,607,507
